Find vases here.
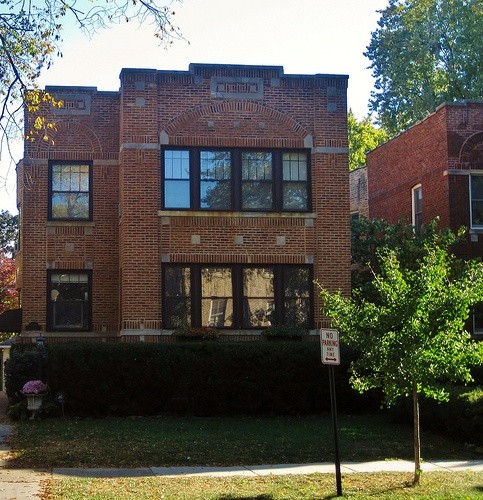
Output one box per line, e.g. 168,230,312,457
24,393,45,421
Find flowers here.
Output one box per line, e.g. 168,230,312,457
20,379,48,394
